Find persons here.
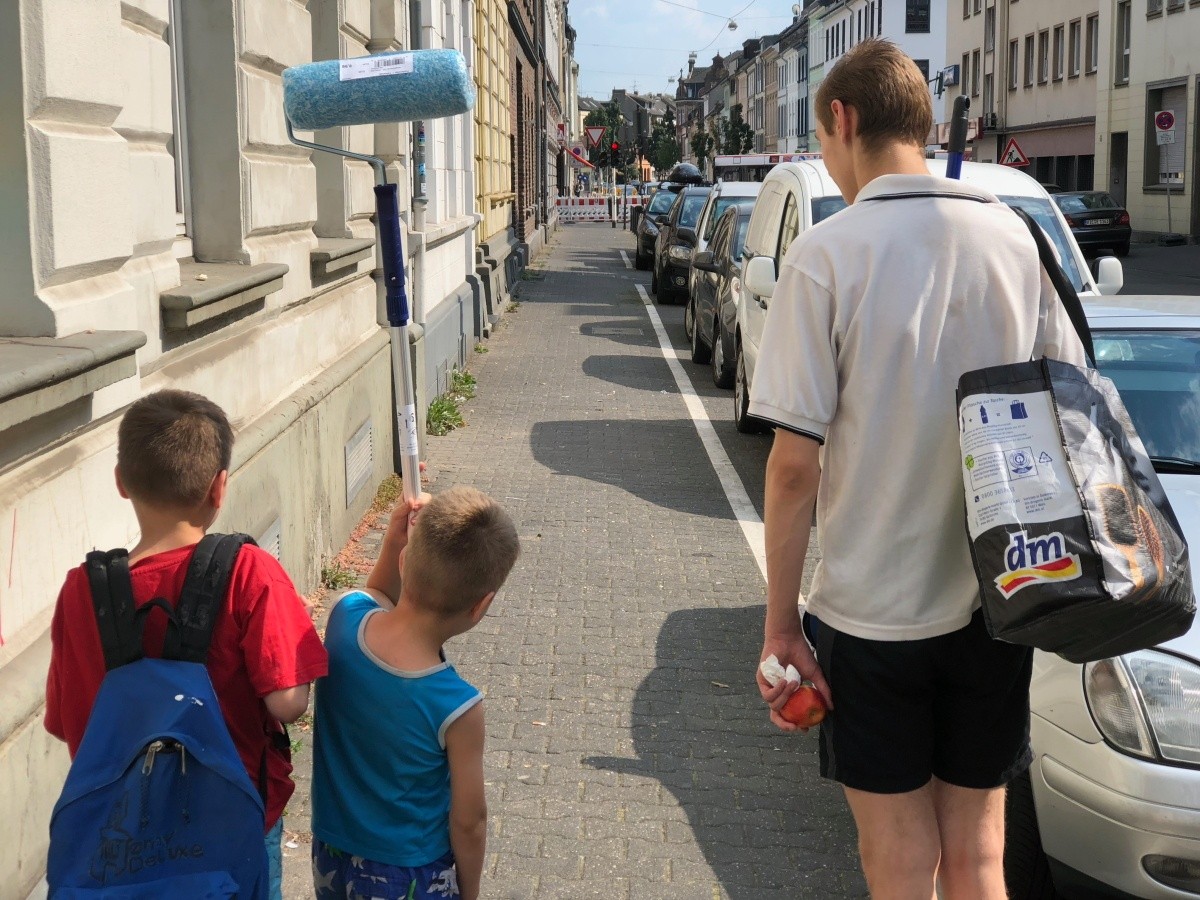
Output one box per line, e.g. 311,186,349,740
43,389,327,900
748,38,1088,899
576,182,583,196
312,462,520,900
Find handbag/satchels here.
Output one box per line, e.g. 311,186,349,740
954,204,1194,664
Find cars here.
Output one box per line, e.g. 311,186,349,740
594,179,661,195
635,180,692,272
1026,292,1200,900
1051,190,1133,256
651,186,712,304
691,201,754,387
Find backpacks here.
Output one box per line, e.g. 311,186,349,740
47,531,268,900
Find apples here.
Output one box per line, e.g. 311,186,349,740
777,684,827,729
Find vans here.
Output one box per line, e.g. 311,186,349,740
734,157,1135,472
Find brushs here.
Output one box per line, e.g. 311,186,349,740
281,45,477,542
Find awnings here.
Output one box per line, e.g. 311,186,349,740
564,147,594,167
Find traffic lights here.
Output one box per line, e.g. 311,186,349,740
599,150,607,167
611,142,620,167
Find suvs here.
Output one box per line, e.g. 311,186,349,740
685,178,763,344
